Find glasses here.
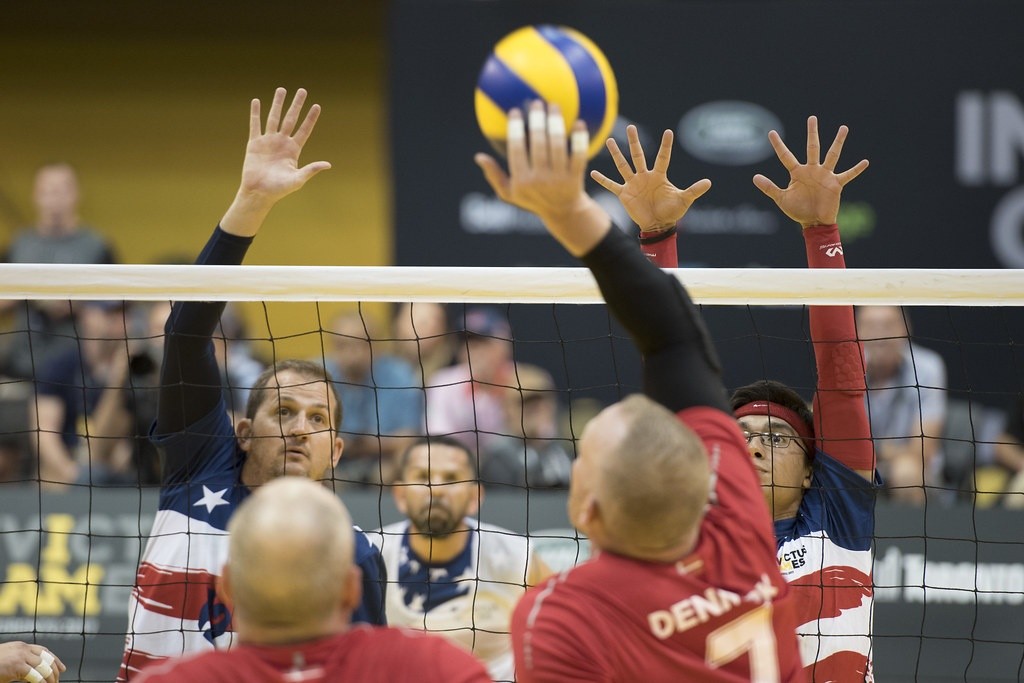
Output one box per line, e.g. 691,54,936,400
740,429,808,454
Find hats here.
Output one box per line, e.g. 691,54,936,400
507,373,552,402
457,311,509,340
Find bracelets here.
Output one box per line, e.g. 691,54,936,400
638,226,678,244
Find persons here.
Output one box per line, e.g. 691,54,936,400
476,100,807,683
117,85,388,683
938,396,1023,508
853,306,948,511
591,114,877,682
1,159,583,493
0,640,67,683
364,432,551,683
125,477,494,683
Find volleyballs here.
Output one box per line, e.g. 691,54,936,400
474,22,620,163
